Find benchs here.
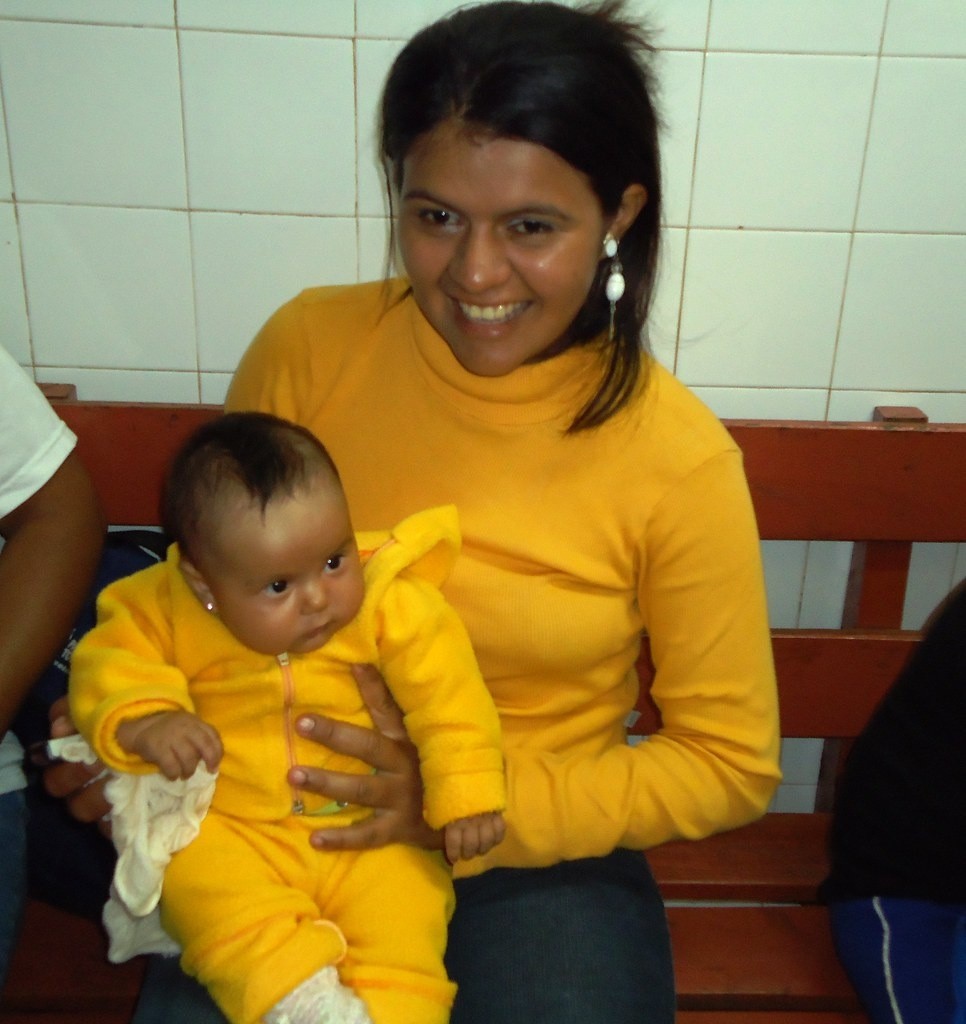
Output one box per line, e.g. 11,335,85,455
0,377,966,1024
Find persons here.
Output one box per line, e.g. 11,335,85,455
0,344,100,980
37,0,780,1024
826,580,966,1024
67,412,504,1024
